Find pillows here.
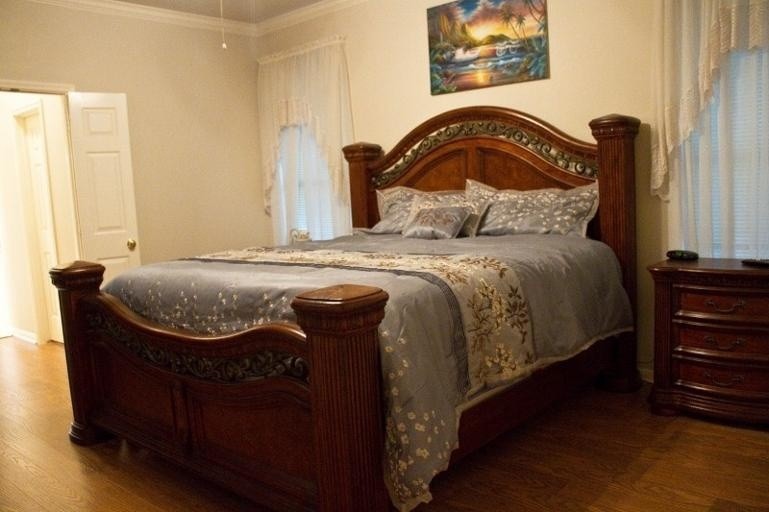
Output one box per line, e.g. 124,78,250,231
361,179,600,240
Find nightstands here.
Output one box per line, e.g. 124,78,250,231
648,251,768,430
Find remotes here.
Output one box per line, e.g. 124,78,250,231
742,259,769,268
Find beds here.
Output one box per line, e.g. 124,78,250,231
49,106,639,512
